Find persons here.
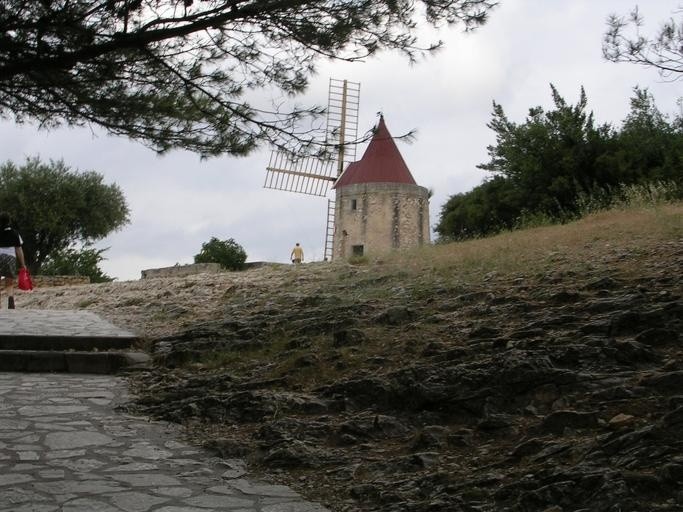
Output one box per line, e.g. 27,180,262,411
0,213,27,308
290,243,304,264
